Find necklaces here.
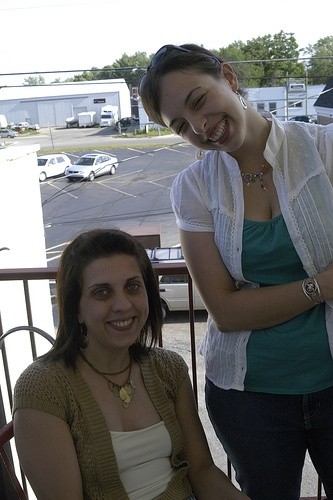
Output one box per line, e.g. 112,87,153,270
242,163,270,190
79,350,135,409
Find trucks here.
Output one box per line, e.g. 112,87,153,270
99,105,119,127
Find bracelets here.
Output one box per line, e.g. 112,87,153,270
302,276,323,304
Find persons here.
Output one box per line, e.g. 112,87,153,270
139,45,333,500
12,228,253,500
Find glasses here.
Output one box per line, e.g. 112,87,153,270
137,45,221,97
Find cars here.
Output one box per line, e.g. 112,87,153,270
37,154,73,182
64,152,119,182
290,115,318,124
0,128,16,139
15,122,31,129
